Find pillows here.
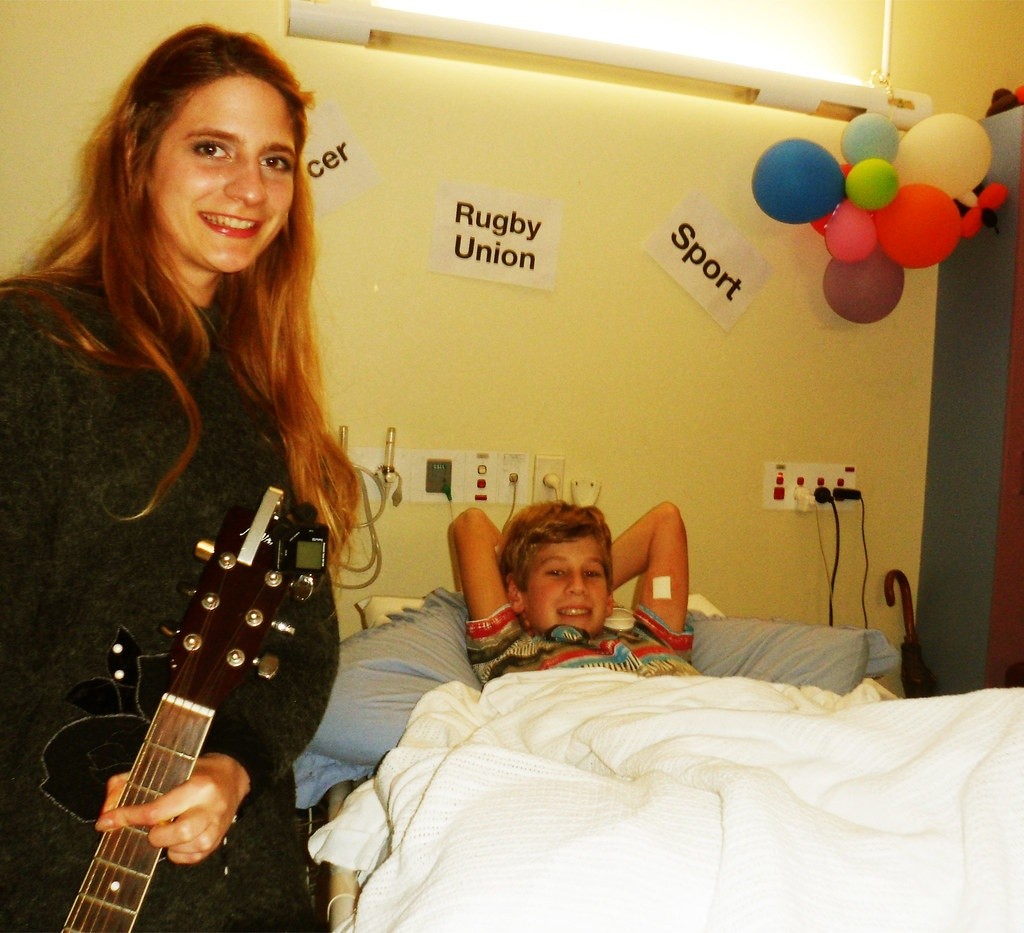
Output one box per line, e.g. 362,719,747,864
292,586,484,809
682,609,902,696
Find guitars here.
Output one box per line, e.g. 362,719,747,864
58,482,328,932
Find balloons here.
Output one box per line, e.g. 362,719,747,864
752,112,1007,323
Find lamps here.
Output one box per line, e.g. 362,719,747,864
287,0,933,133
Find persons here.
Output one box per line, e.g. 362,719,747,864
0,24,360,933
454,500,699,686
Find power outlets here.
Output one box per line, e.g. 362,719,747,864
765,461,858,511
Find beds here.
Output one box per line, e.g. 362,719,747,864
293,591,1024,933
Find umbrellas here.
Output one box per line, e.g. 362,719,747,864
885,569,939,698
